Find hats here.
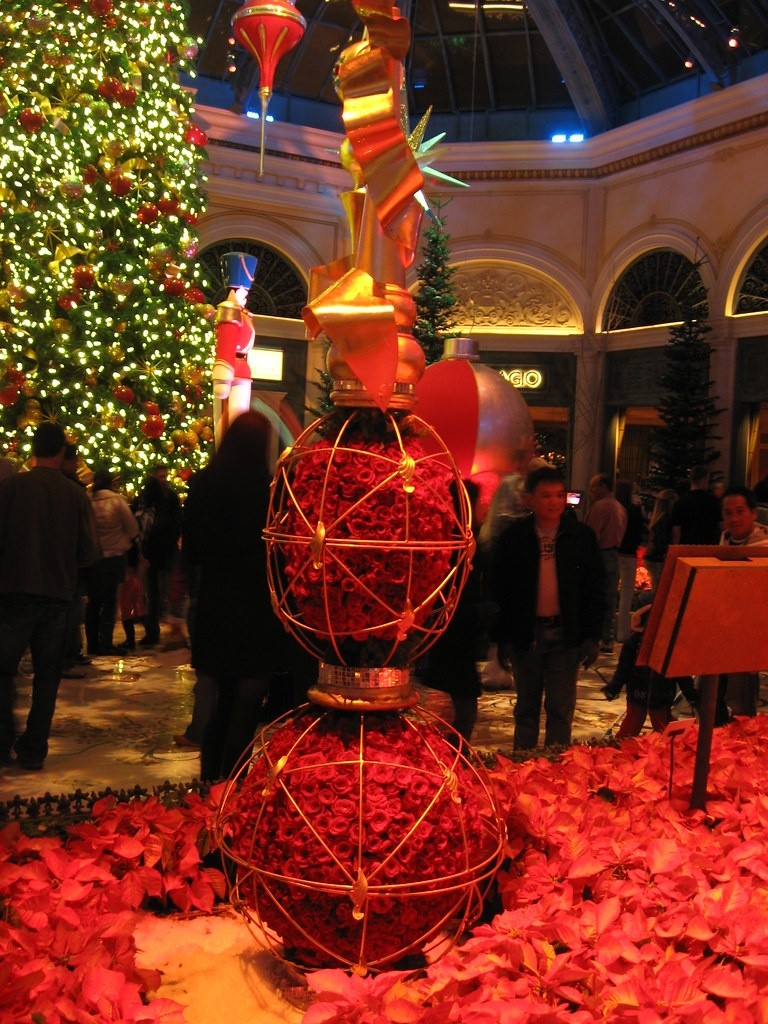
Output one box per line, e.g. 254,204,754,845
652,489,679,503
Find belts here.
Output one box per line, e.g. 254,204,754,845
601,546,618,550
537,615,560,626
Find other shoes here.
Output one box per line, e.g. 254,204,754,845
173,734,201,746
139,637,155,645
86,641,109,656
71,654,92,664
117,639,136,649
598,646,614,655
61,666,87,678
16,754,44,769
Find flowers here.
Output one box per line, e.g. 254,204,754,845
296,710,768,1024
236,717,490,965
0,775,238,1024
287,429,454,649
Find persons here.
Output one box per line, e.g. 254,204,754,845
488,467,612,752
55,440,768,692
600,591,701,740
0,420,104,772
697,484,767,726
179,410,295,782
413,475,495,757
